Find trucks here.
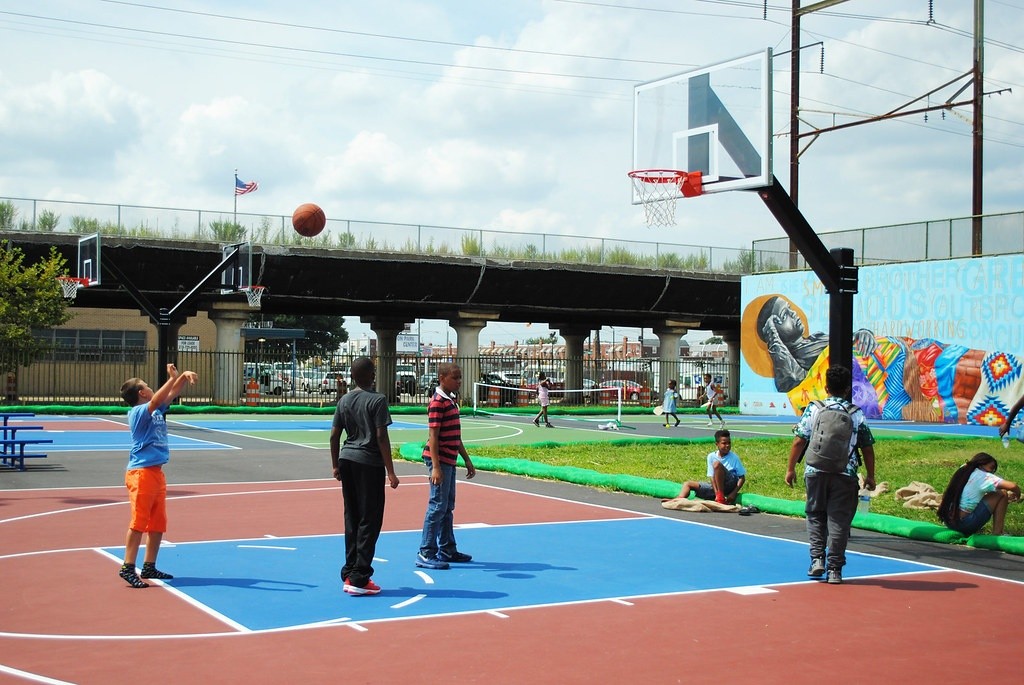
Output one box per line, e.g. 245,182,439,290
397,364,417,394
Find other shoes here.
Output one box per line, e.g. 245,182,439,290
532,418,540,427
545,422,554,428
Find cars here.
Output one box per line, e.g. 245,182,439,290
244,362,352,396
582,379,598,397
416,372,440,399
599,380,658,401
480,371,521,405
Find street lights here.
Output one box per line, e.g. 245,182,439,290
418,330,439,356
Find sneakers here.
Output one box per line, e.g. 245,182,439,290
806,557,826,577
347,578,382,596
826,569,842,584
415,551,450,569
435,548,472,562
343,575,375,592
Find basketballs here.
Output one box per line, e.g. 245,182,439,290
292,203,325,237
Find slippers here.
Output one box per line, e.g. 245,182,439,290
739,503,761,517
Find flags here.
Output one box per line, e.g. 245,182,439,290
236,175,258,196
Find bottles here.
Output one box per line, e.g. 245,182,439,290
1001,432,1011,448
860,487,870,520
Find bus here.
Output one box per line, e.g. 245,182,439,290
523,366,565,384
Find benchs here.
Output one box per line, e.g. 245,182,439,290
0,413,53,471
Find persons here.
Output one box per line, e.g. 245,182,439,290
330,357,399,596
119,363,199,589
784,364,876,584
532,371,556,428
936,451,1024,535
659,430,745,505
998,394,1024,438
415,363,475,569
660,380,683,427
699,374,726,429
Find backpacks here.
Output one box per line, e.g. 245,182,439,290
804,400,862,473
